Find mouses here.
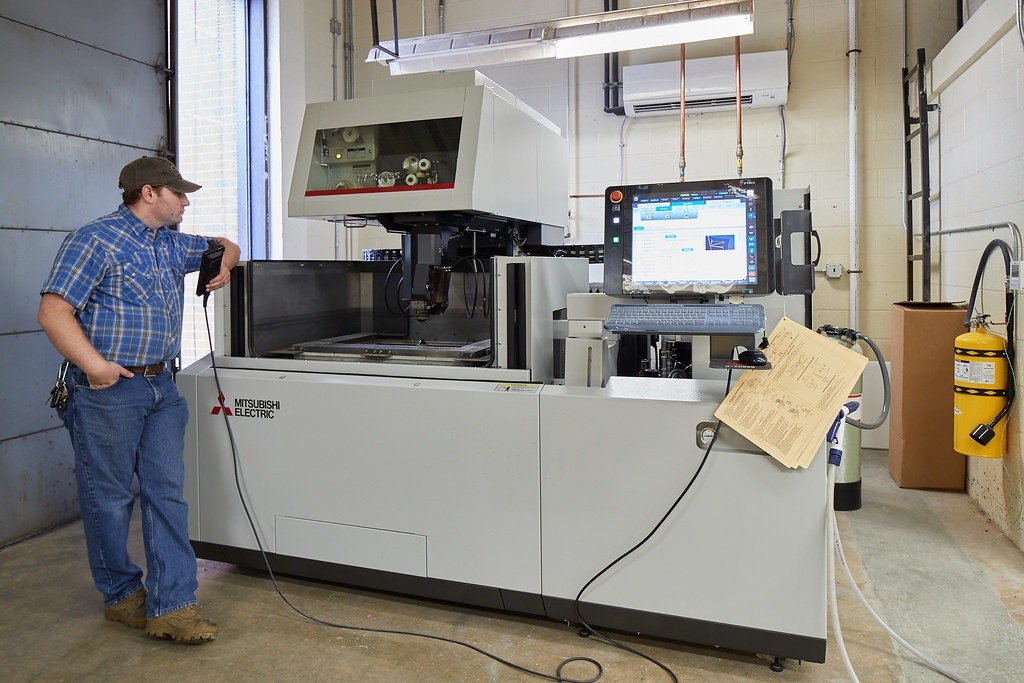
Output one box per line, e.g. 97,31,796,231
738,350,767,366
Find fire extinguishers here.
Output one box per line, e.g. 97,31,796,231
817,324,892,511
954,239,1016,457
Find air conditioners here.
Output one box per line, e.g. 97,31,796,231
621,50,789,117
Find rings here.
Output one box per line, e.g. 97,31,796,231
222,283,226,286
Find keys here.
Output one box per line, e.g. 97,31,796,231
44,387,56,405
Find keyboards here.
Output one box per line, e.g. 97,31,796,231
603,304,766,334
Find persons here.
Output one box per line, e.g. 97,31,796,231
38,156,240,643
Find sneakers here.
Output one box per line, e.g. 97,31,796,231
145,604,218,644
104,587,147,629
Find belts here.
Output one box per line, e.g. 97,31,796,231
122,362,164,377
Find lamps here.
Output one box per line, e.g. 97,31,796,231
364,0,755,78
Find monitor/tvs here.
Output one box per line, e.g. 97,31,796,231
601,177,776,299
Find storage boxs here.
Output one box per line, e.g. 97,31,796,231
887,305,968,492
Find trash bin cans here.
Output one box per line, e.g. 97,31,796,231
891,300,971,490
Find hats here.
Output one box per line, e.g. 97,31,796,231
119,156,202,193
